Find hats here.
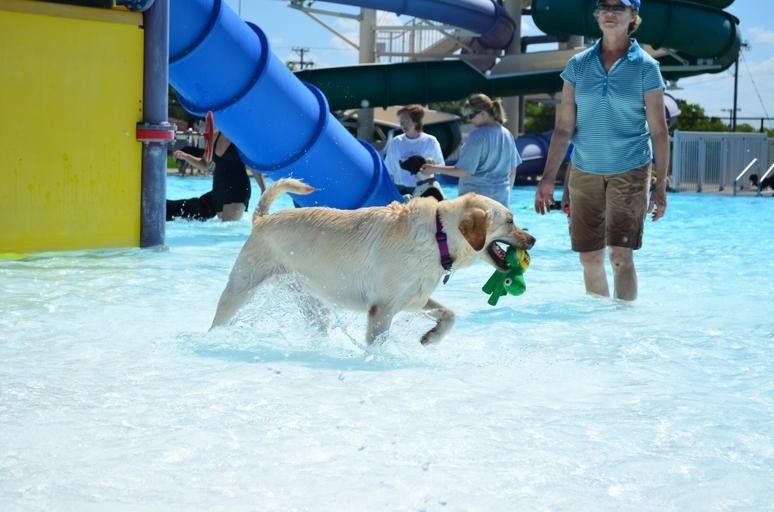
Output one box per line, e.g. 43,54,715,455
620,0,640,12
399,155,426,175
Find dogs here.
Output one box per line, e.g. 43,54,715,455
207,178,537,361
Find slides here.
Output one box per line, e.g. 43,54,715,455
168,0,740,210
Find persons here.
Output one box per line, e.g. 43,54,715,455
173,129,266,223
419,95,523,211
535,1,669,301
382,105,445,201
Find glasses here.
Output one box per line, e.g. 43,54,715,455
466,110,481,119
596,3,625,13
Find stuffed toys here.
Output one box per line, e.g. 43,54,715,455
480,228,531,307
